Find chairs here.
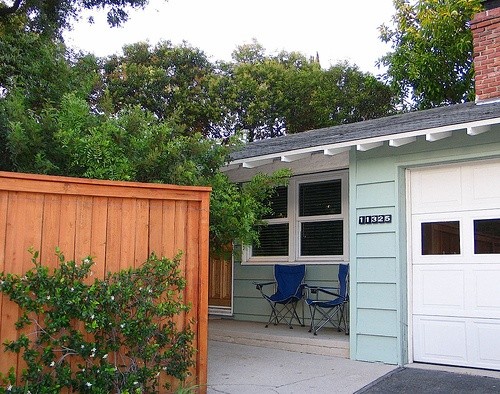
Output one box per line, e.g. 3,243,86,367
252,264,305,329
304,263,349,336
